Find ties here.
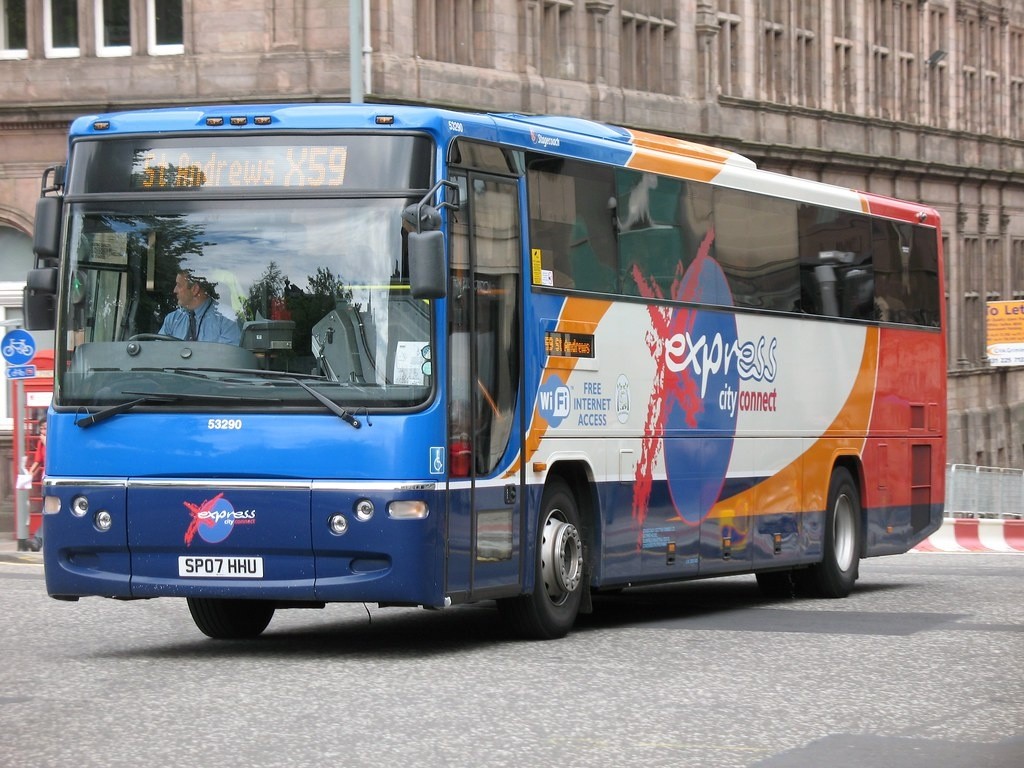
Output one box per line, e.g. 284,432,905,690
185,310,197,341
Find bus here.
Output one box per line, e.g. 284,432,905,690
21,104,949,641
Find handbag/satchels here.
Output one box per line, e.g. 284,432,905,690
15,474,32,490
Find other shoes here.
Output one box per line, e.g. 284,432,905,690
25,537,42,552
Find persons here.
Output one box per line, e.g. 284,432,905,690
155,266,242,346
